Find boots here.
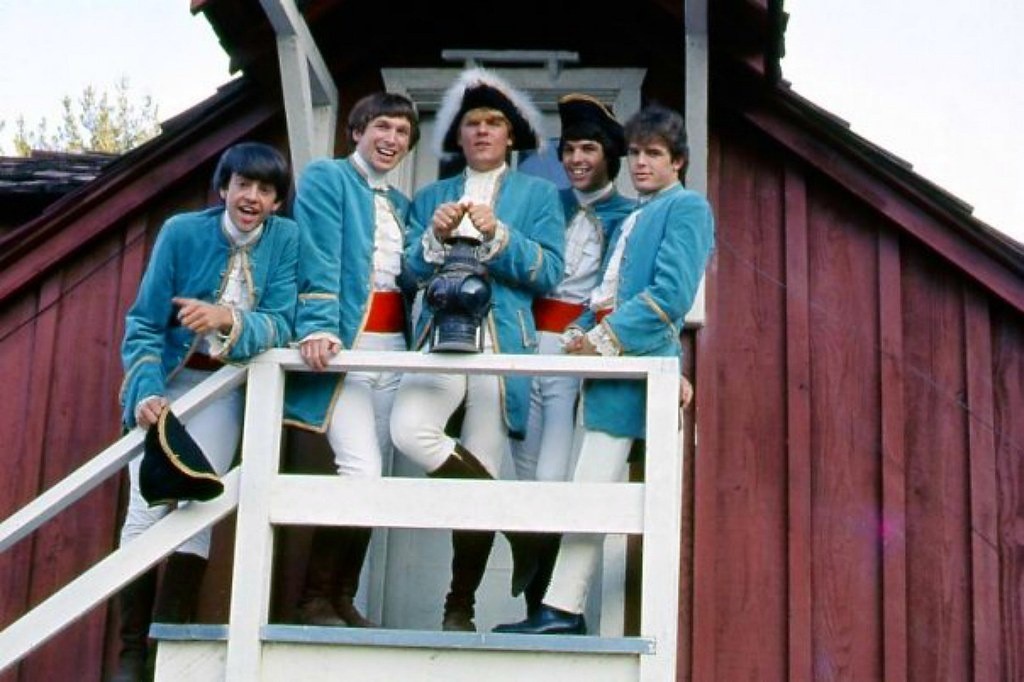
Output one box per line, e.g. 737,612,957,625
297,525,379,627
442,530,499,636
430,444,558,595
153,548,210,624
113,564,159,679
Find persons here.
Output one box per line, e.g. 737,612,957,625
101,141,299,682
493,105,715,635
293,94,421,629
396,62,566,630
510,91,694,622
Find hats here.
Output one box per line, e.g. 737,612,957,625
557,89,631,163
135,405,228,507
437,67,551,163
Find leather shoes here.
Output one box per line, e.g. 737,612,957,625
493,603,588,635
